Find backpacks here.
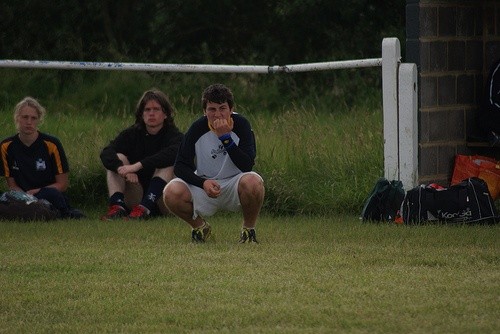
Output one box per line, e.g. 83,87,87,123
362,178,405,223
0,190,60,220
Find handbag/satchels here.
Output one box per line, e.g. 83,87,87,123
403,176,500,225
452,153,500,200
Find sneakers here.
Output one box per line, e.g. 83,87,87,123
100,199,130,221
122,199,161,220
191,218,212,244
239,225,258,244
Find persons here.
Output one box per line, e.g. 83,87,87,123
100,90,184,223
0,96,85,217
163,84,265,246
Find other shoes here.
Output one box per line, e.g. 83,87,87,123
70,208,87,219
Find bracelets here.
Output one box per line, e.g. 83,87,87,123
218,134,236,149
